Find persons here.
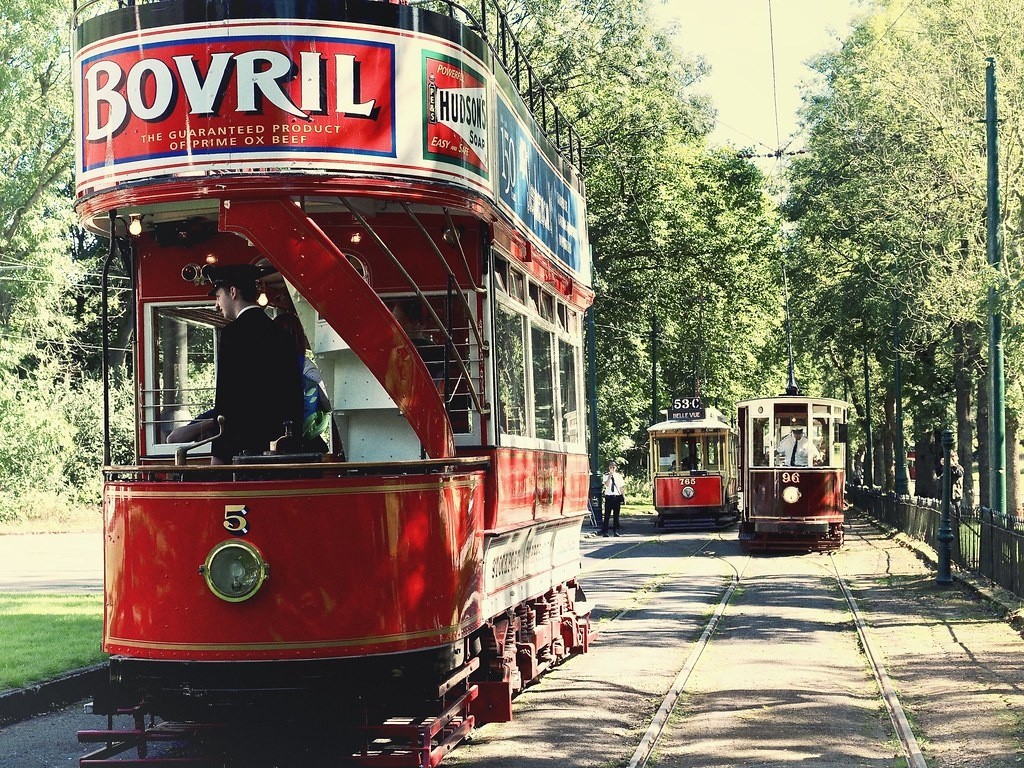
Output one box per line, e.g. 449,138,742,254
852,466,862,486
602,462,625,537
187,260,331,482
764,427,826,466
393,297,445,398
936,447,964,527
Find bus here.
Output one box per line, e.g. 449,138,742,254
68,0,597,768
646,396,741,532
734,395,850,552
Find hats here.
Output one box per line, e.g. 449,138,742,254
201,263,267,297
791,425,803,431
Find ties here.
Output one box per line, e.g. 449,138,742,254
610,475,616,492
791,440,798,466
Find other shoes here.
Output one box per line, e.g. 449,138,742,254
614,531,619,537
603,533,610,537
